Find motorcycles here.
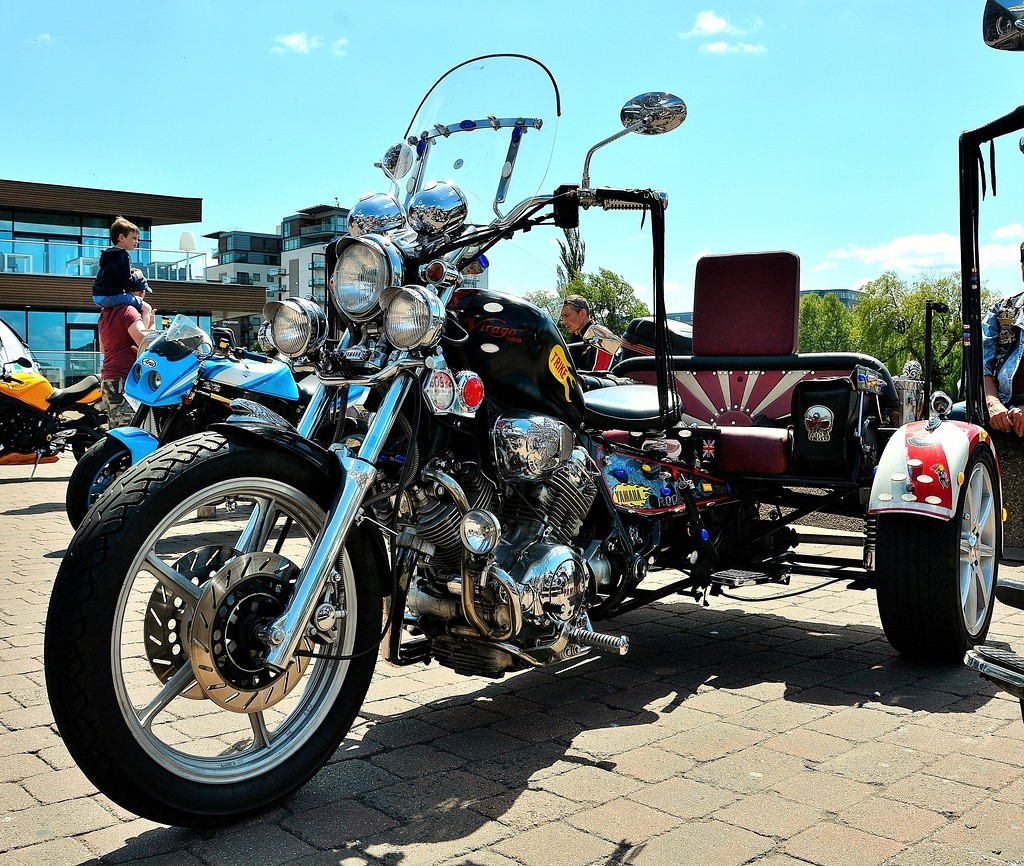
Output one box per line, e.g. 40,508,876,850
0,48,1006,831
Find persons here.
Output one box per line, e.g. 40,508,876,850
90,217,153,354
97,268,158,432
981,240,1024,441
561,293,619,374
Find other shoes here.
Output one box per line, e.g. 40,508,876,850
131,344,139,354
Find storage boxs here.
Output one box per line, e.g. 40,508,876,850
893,380,932,428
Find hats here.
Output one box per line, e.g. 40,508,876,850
132,272,154,293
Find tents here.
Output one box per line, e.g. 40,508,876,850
0,316,59,465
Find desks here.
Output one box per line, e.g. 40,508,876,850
147,261,179,282
4,253,33,274
65,257,98,276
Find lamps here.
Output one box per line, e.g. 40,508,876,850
178,232,196,281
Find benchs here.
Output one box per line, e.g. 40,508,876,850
604,352,902,473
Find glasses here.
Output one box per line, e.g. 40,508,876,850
559,298,585,311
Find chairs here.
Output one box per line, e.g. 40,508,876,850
0,252,18,273
133,262,192,280
